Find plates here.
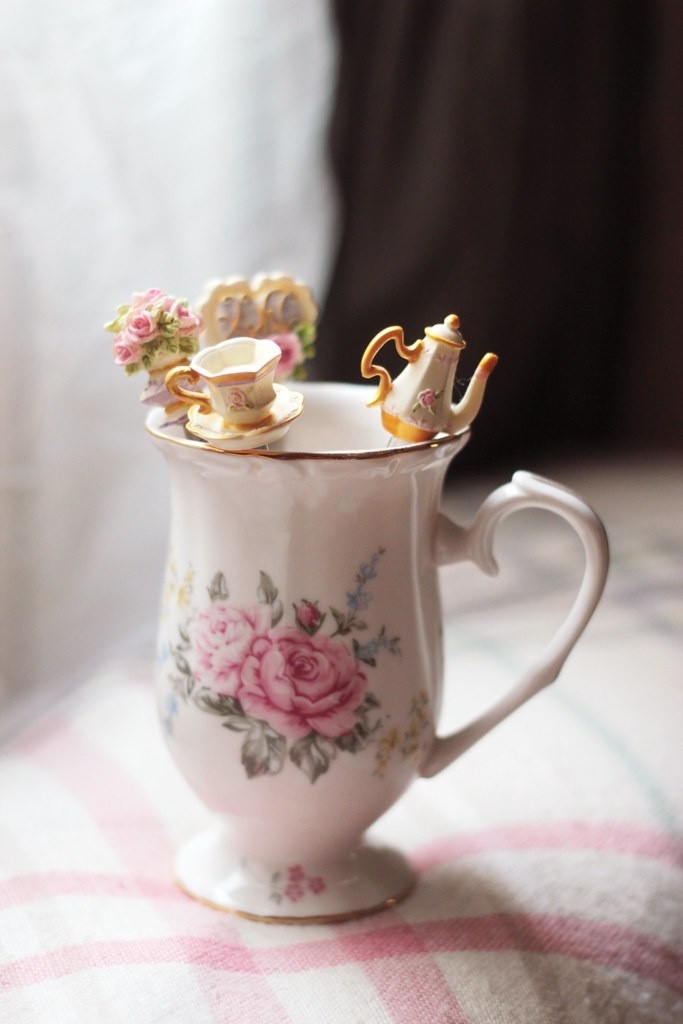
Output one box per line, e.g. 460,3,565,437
179,389,305,450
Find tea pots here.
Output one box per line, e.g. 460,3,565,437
358,315,498,442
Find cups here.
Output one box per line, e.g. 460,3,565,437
146,384,612,924
166,338,281,427
